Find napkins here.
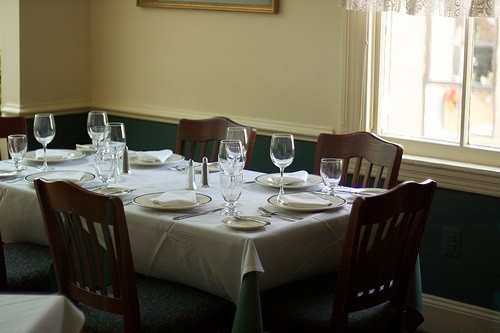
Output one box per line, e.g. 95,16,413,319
36,149,70,158
152,190,196,208
275,170,308,185
39,171,84,182
282,192,333,208
136,148,172,164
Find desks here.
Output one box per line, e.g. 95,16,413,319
0,150,424,333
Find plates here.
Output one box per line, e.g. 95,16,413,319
126,150,184,166
0,147,212,210
222,214,269,230
255,172,325,190
77,144,97,152
22,148,85,162
267,190,346,214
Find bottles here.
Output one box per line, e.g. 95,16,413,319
202,156,211,188
186,158,198,190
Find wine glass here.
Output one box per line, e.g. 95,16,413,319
104,120,127,174
220,124,249,216
33,112,56,170
269,132,295,195
86,111,110,155
320,157,344,196
8,135,28,177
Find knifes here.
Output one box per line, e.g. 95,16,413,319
173,207,224,221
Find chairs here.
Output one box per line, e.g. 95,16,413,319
175,117,256,170
34,178,235,333
280,179,437,333
314,132,404,190
0,116,27,161
